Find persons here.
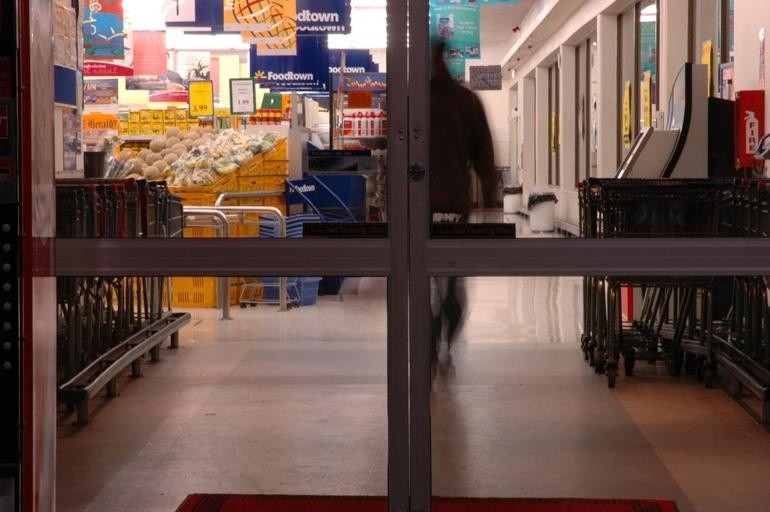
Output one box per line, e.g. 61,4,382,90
406,35,499,379
437,17,451,39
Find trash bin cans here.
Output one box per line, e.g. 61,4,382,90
529,193,555,232
502,185,522,213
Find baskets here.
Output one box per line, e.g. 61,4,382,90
171,277,236,307
238,177,263,192
264,137,288,160
238,275,262,306
167,171,237,206
257,214,323,305
238,153,264,176
183,227,216,238
264,161,289,175
264,176,288,191
265,196,285,216
238,197,263,219
228,220,259,237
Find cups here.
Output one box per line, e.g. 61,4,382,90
83,150,108,179
652,111,666,131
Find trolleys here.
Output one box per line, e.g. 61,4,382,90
55,174,187,382
579,174,768,384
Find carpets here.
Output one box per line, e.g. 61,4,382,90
174,493,677,512
